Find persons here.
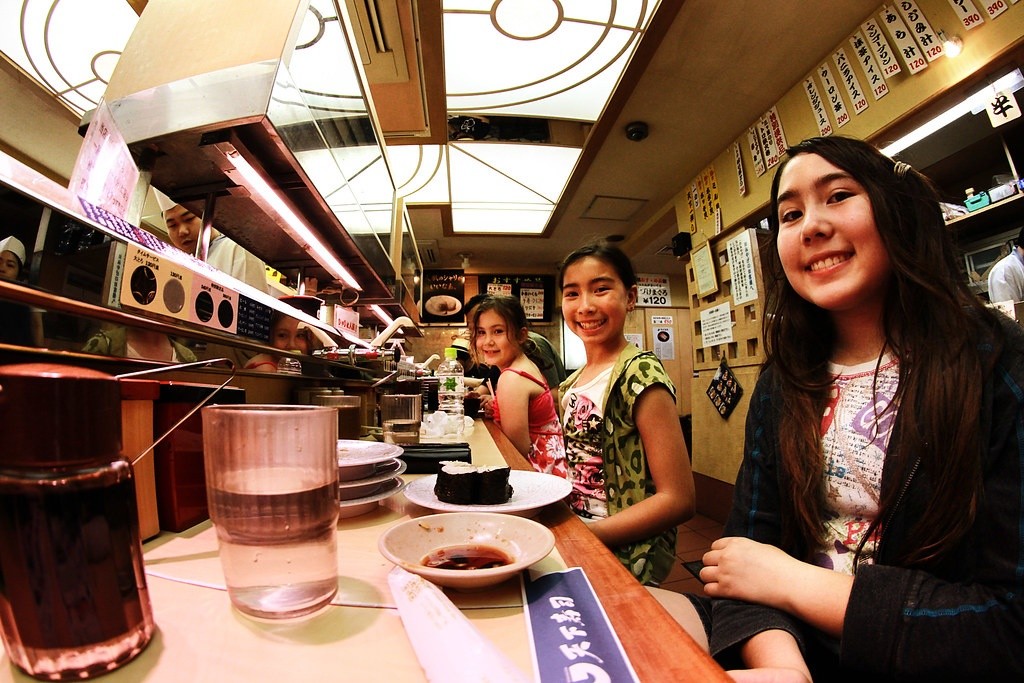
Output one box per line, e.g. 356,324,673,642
243,310,312,372
0,236,47,346
468,294,567,478
698,137,1024,683
464,294,567,389
81,325,198,362
556,240,696,587
988,227,1024,302
153,186,270,295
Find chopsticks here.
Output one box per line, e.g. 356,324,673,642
486,378,494,399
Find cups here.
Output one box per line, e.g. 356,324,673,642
201,404,340,619
380,394,421,446
316,395,361,441
385,359,416,381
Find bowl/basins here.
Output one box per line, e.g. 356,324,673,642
378,512,555,593
464,397,480,420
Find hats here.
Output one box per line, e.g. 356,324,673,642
152,187,177,212
0,236,26,265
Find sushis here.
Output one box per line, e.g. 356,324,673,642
434,460,514,505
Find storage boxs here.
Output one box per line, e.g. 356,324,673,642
963,191,990,212
988,182,1018,204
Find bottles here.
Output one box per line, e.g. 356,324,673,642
276,356,301,375
0,356,156,683
437,348,464,433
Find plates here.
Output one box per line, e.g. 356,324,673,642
368,430,384,441
425,295,462,316
338,439,407,519
403,470,573,518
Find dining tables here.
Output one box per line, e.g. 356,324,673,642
0,418,735,683
